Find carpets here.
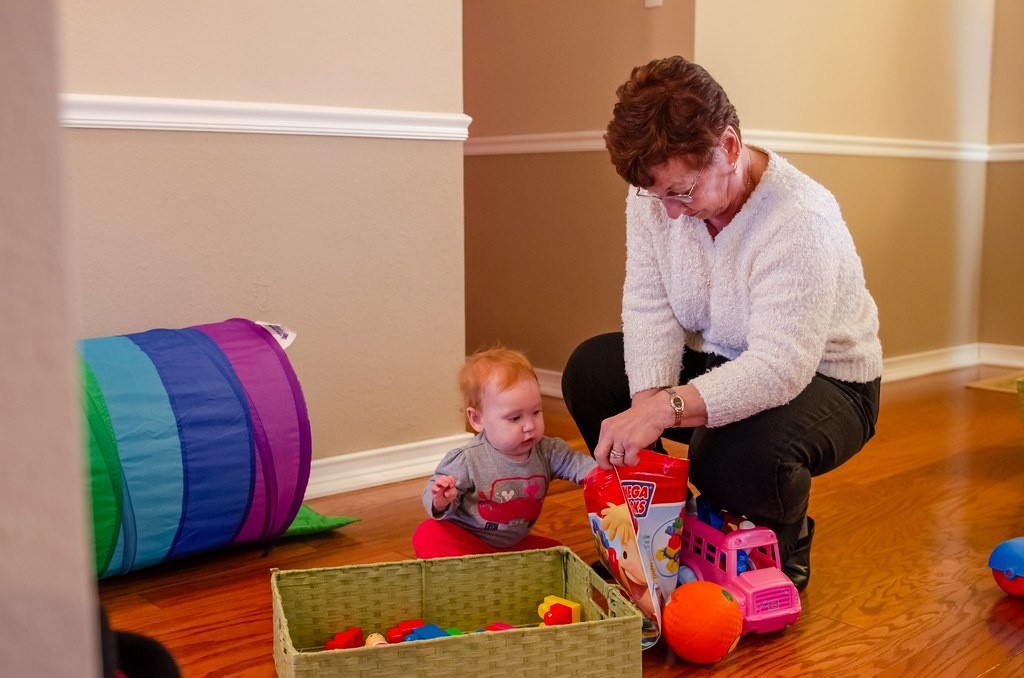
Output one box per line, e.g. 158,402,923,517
967,369,1024,396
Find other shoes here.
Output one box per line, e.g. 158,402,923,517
779,519,814,589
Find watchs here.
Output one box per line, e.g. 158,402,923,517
665,388,685,428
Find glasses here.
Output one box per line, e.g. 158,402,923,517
636,143,717,203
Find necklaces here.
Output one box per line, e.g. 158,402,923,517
713,154,751,237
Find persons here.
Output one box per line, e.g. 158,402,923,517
561,56,883,594
411,344,600,560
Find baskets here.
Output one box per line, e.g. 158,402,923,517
272,548,641,678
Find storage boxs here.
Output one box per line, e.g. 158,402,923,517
272,543,644,678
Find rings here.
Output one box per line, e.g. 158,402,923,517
610,453,621,458
611,449,624,455
